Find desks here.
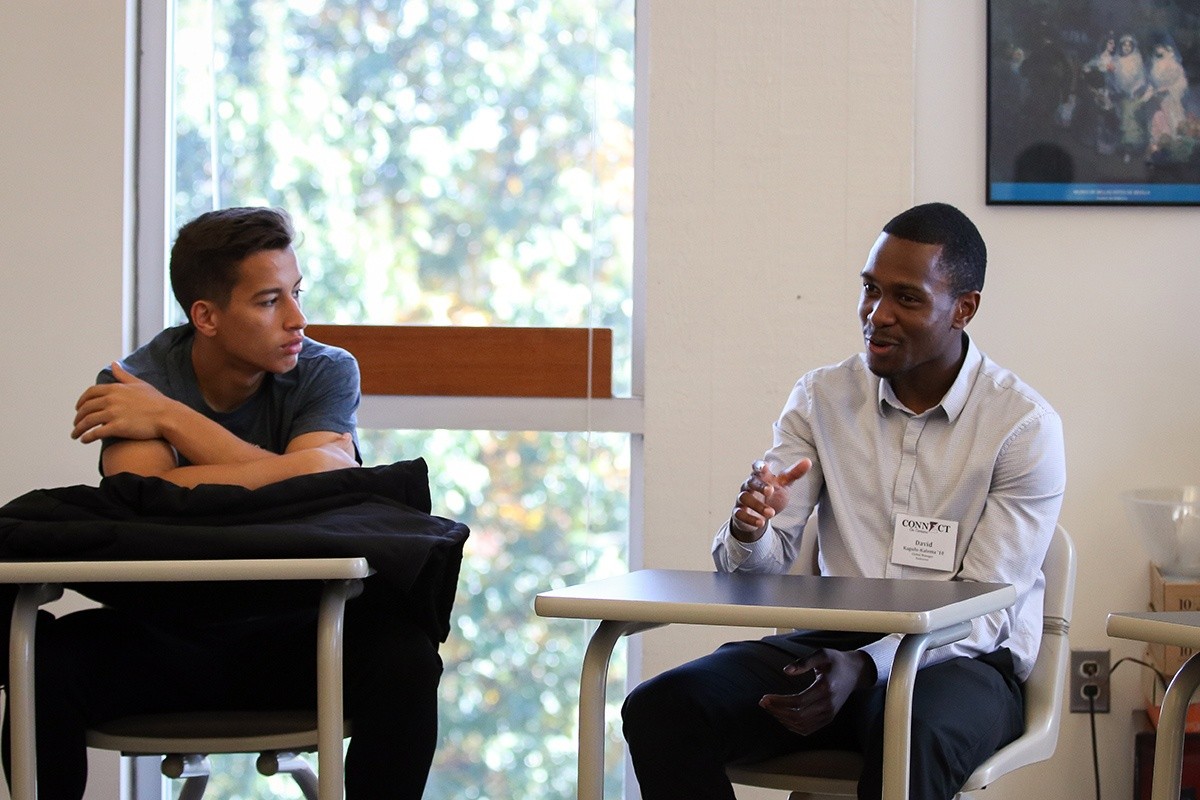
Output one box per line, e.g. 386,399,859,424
535,570,1016,800
1107,610,1200,800
0,548,369,800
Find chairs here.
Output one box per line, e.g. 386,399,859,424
79,709,355,800
724,519,1076,800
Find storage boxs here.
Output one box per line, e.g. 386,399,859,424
1142,561,1199,737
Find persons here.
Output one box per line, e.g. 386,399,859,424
0,206,440,800
621,203,1065,800
1003,32,1188,162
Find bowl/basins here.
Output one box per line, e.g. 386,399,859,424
1118,485,1199,578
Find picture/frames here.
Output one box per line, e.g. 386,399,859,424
985,1,1200,207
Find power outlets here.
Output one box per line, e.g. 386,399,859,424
1067,650,1113,714
